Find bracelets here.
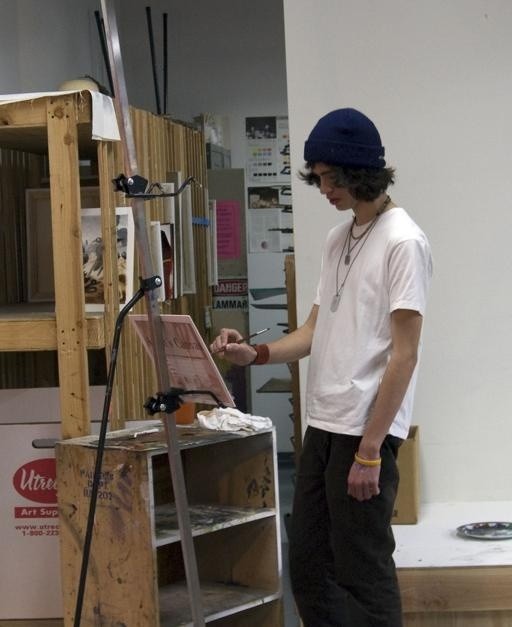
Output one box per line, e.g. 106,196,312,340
251,342,269,365
353,453,382,467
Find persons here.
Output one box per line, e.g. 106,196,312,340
210,108,435,626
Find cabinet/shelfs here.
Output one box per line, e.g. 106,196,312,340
56,421,287,625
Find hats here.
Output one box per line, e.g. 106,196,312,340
304,108,386,168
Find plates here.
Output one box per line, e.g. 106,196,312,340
458,521,512,539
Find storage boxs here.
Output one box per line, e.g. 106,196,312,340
0,384,115,624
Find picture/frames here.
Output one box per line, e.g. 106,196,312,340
24,186,100,303
78,207,136,312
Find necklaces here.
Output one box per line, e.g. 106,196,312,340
329,195,391,312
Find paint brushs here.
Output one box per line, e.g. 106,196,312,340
209,321,270,355
